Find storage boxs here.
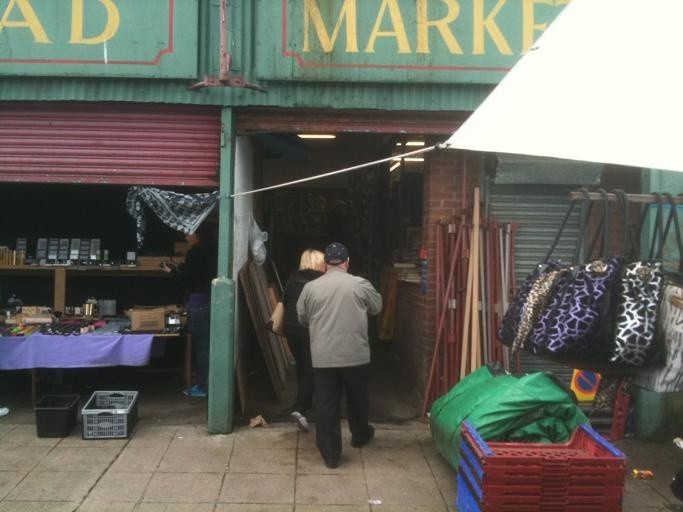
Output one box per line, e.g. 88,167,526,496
130,304,165,332
33,394,80,438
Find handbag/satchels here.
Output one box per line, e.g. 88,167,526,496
263,301,285,337
494,256,683,393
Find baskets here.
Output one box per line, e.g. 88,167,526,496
80,391,139,439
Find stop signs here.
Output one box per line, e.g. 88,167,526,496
570,367,601,401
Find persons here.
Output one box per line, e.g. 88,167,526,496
295,242,383,468
159,231,210,397
281,249,327,434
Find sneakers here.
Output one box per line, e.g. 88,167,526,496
182,384,207,398
289,411,310,433
351,424,374,446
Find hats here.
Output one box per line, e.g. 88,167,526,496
324,240,348,265
